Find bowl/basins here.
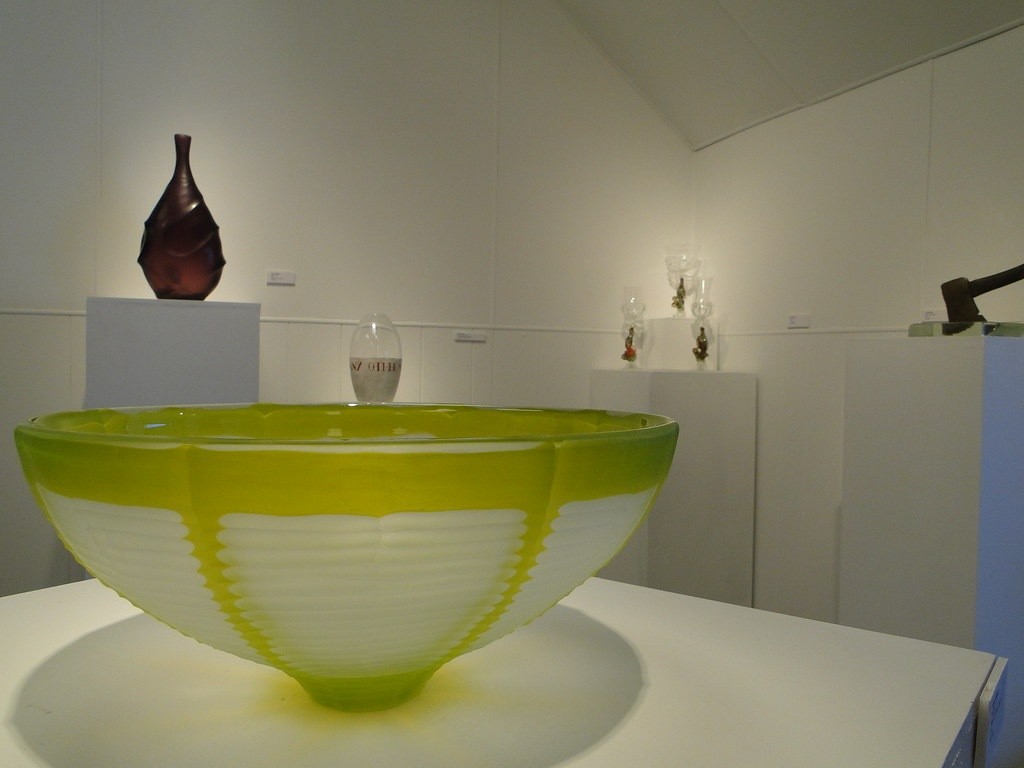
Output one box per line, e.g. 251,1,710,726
14,409,678,712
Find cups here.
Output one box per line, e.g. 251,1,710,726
347,312,402,404
